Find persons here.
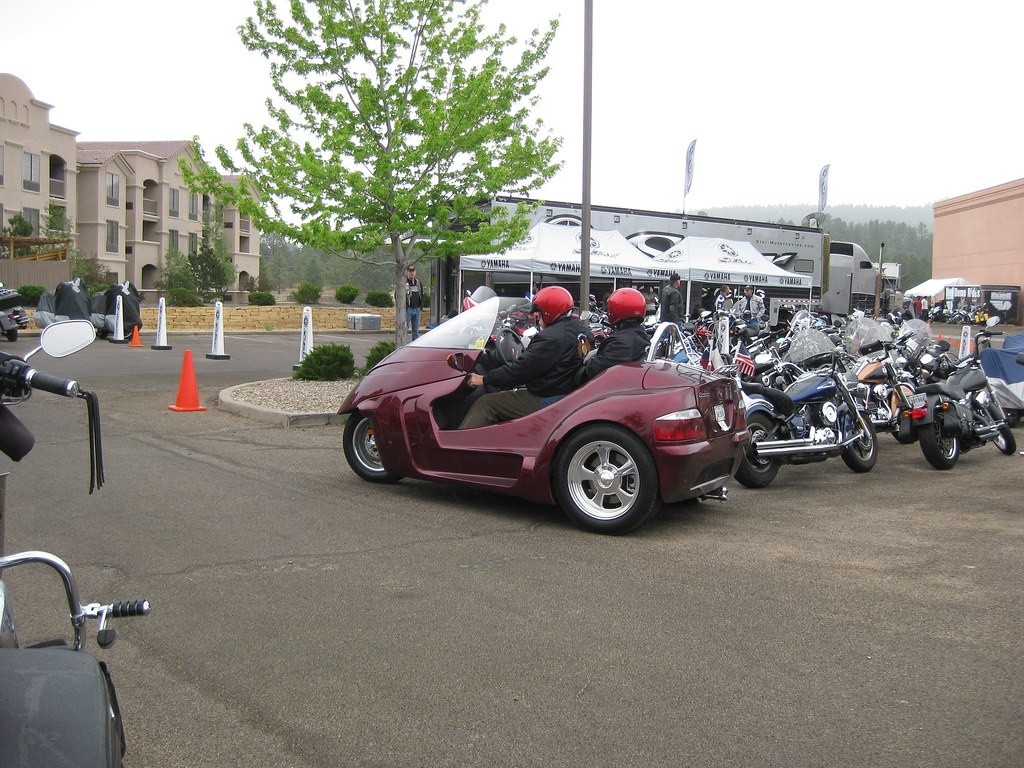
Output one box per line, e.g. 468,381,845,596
405,266,424,340
457,287,595,429
603,285,614,311
715,285,730,312
913,296,928,318
660,273,684,344
538,288,652,409
525,283,538,301
738,285,765,321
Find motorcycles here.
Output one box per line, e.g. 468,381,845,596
429,283,960,445
907,315,1018,468
336,293,751,535
928,300,990,327
0,282,30,342
728,320,878,489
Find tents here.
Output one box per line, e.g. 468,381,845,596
654,236,813,330
904,278,963,297
458,223,693,323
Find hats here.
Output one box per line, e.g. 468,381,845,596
743,286,753,290
407,264,416,271
669,273,680,280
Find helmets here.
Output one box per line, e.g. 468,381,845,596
607,287,646,326
532,285,574,326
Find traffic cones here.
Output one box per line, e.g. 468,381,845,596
128,325,145,347
167,350,208,411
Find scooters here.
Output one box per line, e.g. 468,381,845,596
0,321,150,768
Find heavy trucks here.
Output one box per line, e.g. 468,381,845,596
428,195,882,343
